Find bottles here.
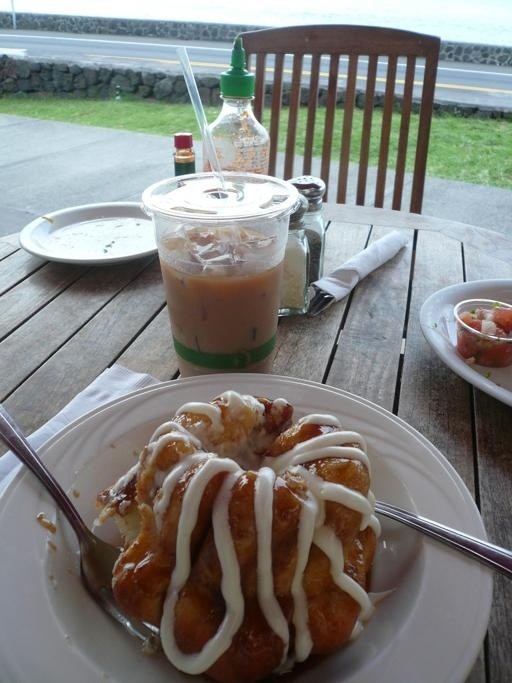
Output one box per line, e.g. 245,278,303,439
173,132,197,176
202,37,271,176
278,195,310,317
285,175,326,284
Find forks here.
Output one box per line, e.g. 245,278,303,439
302,284,334,321
1,402,160,644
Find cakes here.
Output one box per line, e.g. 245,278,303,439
100,388,374,675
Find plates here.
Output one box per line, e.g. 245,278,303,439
0,373,494,683
419,278,512,407
18,202,157,265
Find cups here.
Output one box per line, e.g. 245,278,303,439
141,170,300,376
454,299,512,369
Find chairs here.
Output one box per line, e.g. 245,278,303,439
233,25,440,217
